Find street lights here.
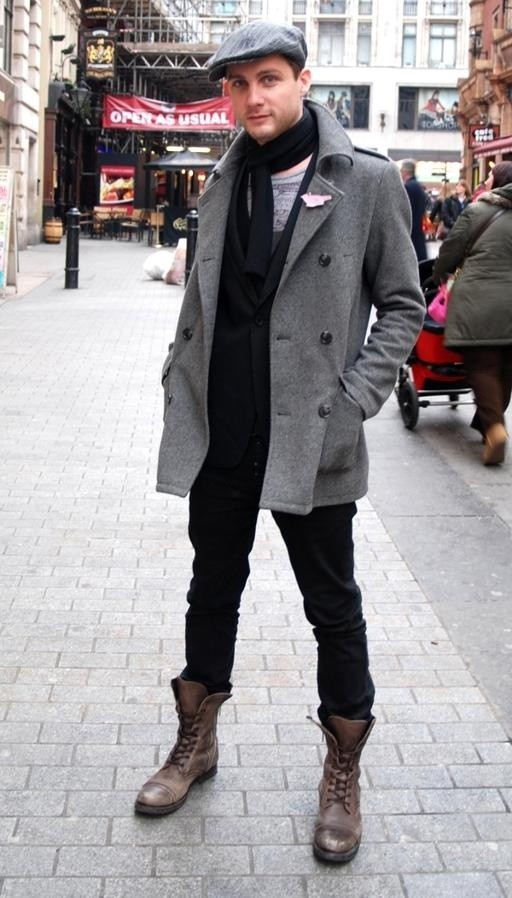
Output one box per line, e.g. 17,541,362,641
64,77,92,288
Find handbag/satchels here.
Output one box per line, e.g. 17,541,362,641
428,272,456,323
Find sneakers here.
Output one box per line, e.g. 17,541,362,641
482,423,508,465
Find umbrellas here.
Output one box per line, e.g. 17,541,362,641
143,147,221,209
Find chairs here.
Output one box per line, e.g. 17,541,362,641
92,205,156,243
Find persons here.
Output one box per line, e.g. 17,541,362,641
400,159,487,263
324,90,350,128
135,18,426,865
425,88,459,129
432,160,512,466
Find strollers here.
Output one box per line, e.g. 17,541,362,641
396,258,478,430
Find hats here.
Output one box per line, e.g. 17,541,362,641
207,21,309,83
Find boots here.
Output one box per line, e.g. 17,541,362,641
307,709,377,867
133,677,234,818
